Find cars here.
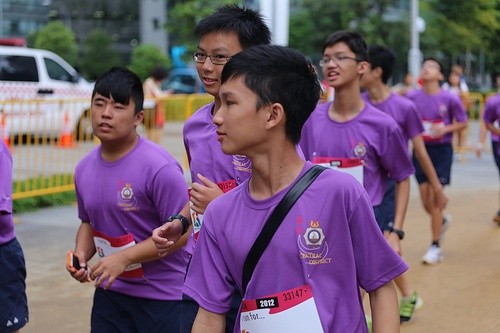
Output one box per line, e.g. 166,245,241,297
160,69,207,95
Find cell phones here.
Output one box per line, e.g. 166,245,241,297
67,250,87,271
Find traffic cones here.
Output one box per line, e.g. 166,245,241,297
0,114,11,153
56,111,75,148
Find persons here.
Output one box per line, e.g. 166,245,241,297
483,94,500,227
182,42,407,333
65,65,196,333
142,68,172,110
405,59,468,267
442,65,470,153
396,71,411,97
0,138,29,333
66,4,450,333
476,73,500,158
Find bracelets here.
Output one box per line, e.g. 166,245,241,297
387,226,404,241
169,213,189,236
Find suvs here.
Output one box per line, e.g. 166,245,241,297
0,46,95,142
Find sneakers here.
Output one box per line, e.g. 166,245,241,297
440,214,451,237
493,211,500,224
422,244,445,265
399,290,423,324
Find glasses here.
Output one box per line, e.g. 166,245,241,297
319,54,361,66
192,50,232,66
